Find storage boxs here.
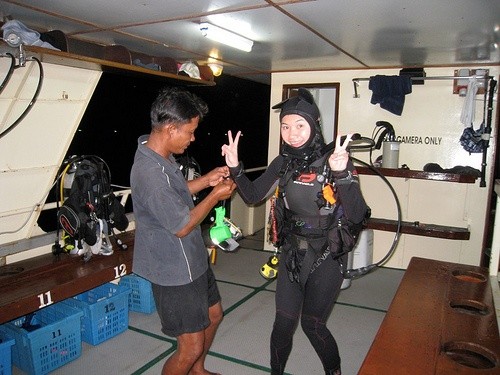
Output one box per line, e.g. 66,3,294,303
0,273,158,375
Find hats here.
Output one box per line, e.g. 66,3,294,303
178,63,201,79
272,88,326,160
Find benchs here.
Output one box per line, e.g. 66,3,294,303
0,228,137,325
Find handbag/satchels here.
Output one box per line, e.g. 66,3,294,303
459,122,485,153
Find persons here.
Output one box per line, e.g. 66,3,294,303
221,89,372,375
130,87,237,375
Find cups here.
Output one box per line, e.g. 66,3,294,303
382,140,400,169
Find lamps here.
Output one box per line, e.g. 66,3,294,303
207,63,224,77
198,22,254,53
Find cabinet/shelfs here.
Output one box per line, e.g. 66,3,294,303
353,165,475,241
